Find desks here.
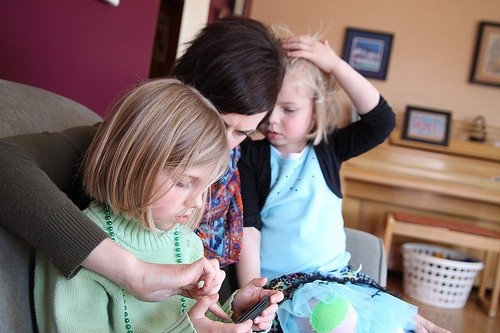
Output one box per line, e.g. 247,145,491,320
339,145,500,287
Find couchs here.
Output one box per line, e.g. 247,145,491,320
0,79,386,333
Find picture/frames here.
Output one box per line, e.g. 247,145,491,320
342,28,394,81
467,20,500,87
402,105,452,146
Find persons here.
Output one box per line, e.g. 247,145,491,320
34,78,284,333
237,33,451,333
0,14,286,333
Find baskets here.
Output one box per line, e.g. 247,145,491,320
402,243,484,310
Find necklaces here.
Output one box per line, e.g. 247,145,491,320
105,203,187,333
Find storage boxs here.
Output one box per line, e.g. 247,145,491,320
402,242,484,310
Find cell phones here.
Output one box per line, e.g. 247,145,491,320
234,295,270,325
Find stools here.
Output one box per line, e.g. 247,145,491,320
381,211,500,317
